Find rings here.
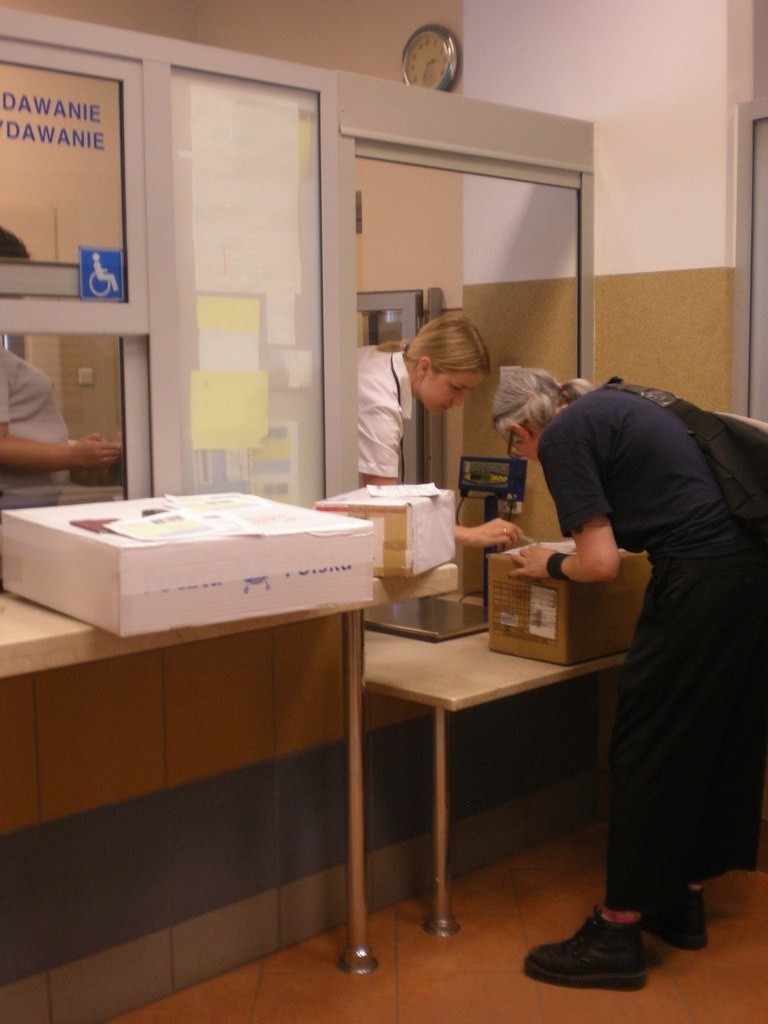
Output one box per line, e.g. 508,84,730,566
504,528,506,534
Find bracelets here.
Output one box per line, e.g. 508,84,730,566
546,552,573,581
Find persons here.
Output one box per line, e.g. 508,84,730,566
355,312,526,549
486,368,768,991
0,226,122,484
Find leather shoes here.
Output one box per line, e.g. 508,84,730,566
524,905,645,992
640,886,705,950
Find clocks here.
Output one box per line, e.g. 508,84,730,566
400,25,459,91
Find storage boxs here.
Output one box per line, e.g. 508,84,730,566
0,494,377,641
485,544,652,666
314,486,455,578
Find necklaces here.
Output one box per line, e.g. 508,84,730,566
390,352,405,484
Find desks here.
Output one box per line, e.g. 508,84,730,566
363,594,628,936
0,563,459,977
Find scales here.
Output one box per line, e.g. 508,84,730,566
362,454,530,644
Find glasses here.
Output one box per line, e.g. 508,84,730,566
506,424,527,459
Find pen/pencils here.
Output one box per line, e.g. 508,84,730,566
520,537,539,544
